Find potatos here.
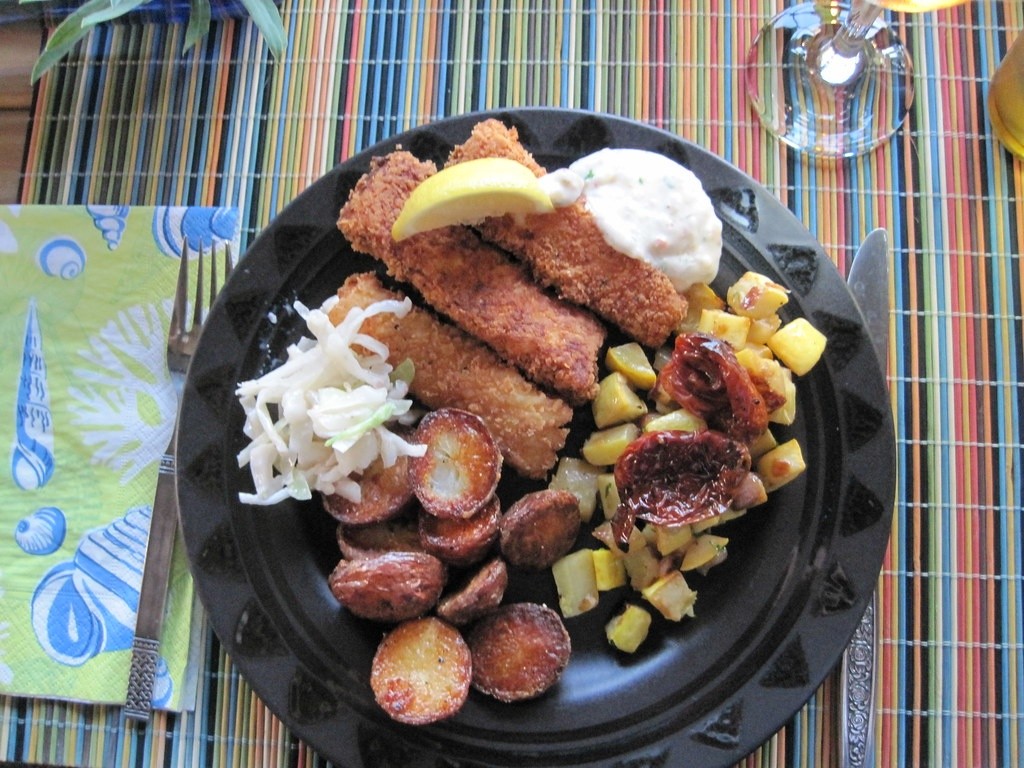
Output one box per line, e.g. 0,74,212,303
315,404,581,724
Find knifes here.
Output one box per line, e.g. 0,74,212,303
845,227,892,385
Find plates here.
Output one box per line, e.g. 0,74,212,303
174,109,896,768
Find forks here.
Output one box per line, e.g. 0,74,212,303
123,239,234,724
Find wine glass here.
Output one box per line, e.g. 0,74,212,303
743,0,917,161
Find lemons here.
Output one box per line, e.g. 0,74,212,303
390,157,555,242
